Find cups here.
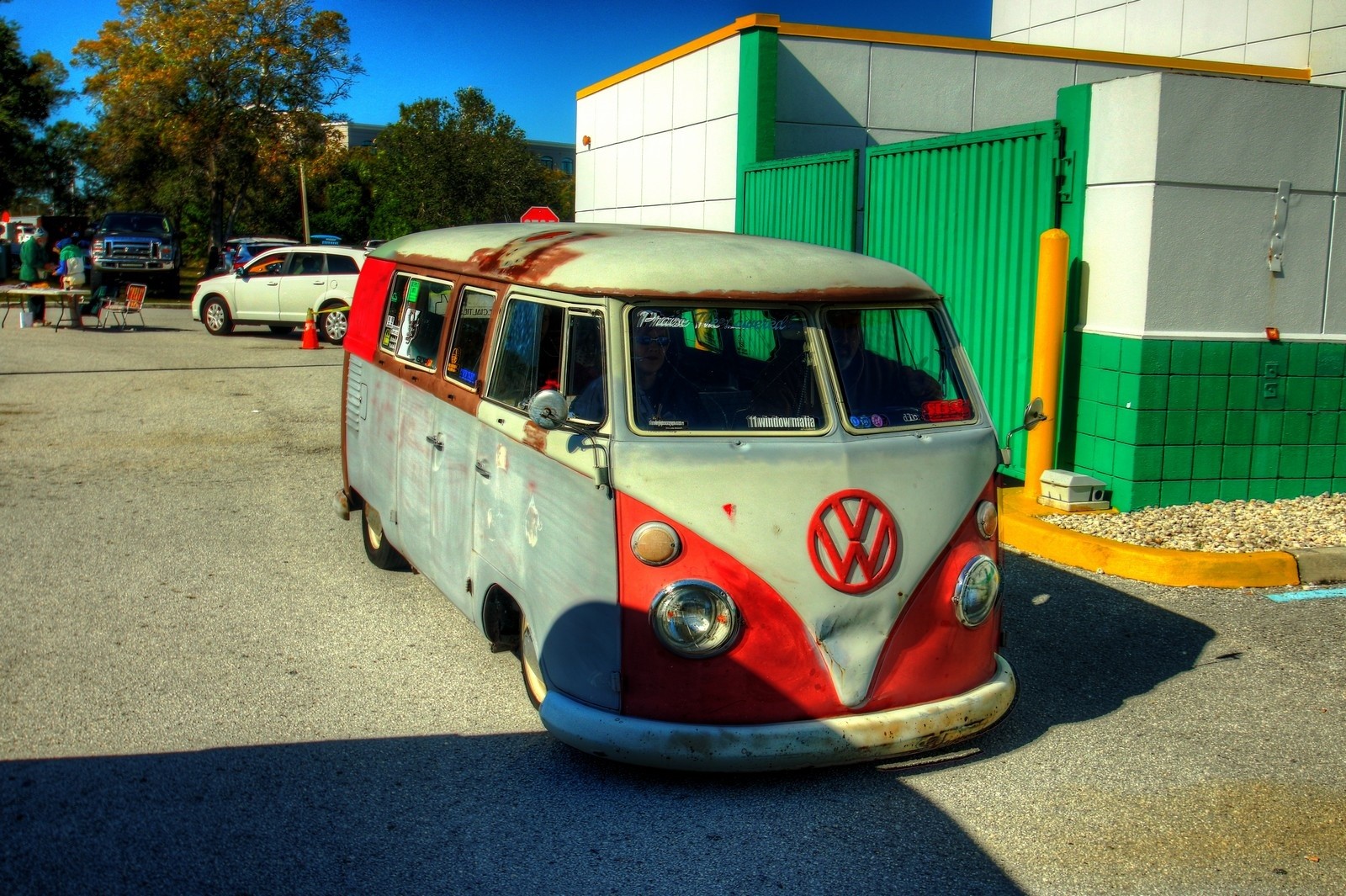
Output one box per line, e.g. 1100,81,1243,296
66,281,71,291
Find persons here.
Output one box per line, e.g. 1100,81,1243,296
572,307,732,430
21,227,86,327
208,246,235,272
755,307,938,427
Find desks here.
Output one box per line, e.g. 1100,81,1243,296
0,285,92,333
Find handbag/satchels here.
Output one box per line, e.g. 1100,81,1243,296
19,312,33,329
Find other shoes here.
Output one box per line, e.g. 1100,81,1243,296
43,319,51,327
32,322,43,328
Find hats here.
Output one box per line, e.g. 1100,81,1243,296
34,227,47,238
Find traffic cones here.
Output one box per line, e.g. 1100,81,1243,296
298,308,323,350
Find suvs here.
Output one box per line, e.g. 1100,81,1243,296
85,210,185,299
189,234,388,346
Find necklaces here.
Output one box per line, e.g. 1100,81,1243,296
797,349,866,416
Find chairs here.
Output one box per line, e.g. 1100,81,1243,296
79,284,147,331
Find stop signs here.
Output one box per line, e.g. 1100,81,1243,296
521,205,559,225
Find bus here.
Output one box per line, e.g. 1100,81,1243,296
331,224,1049,774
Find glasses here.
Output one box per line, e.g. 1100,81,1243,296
635,335,670,348
